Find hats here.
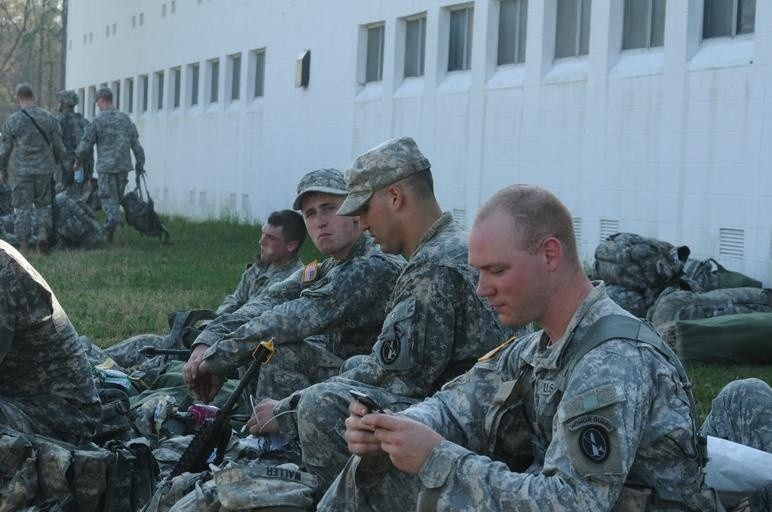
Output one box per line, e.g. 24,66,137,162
335,135,431,217
293,168,349,211
91,88,113,104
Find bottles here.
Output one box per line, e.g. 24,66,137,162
186,404,221,430
73,162,84,184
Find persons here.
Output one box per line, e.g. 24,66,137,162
99,205,308,385
56,92,96,254
186,164,402,418
0,238,160,453
75,86,144,243
247,138,539,512
0,83,62,259
316,179,706,509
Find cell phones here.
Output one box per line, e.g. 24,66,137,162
349,390,385,414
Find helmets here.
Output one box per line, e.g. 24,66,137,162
55,90,79,105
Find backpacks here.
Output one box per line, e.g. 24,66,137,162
646,286,771,330
592,230,691,290
121,166,164,238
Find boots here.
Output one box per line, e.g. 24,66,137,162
36,225,49,257
18,239,31,261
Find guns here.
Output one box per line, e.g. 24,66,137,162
168,337,274,480
